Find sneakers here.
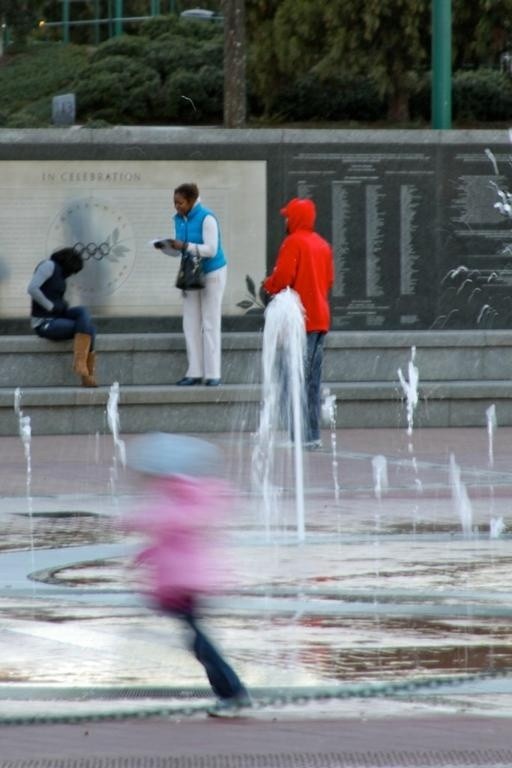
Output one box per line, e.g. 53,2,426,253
287,438,322,451
207,696,255,720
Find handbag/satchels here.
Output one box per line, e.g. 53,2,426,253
176,241,205,289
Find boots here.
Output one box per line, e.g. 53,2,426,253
81,349,98,387
72,332,91,377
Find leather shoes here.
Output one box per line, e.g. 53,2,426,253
206,379,222,385
177,378,201,385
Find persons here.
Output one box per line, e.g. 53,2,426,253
154,180,229,388
258,194,340,450
119,429,260,720
24,245,102,390
